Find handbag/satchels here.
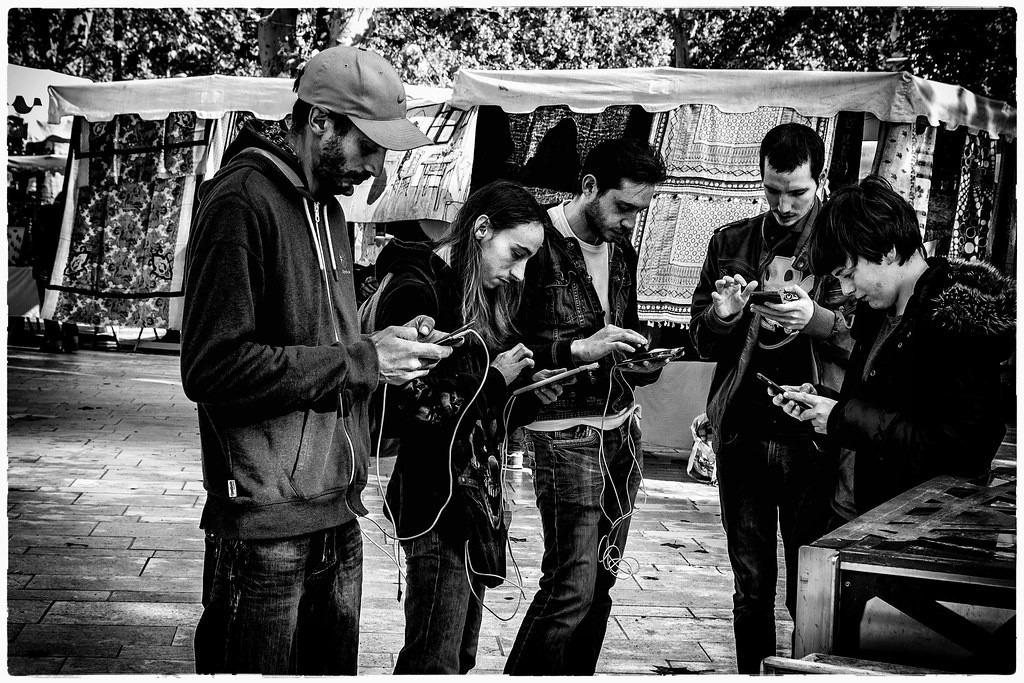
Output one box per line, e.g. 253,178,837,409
457,437,509,589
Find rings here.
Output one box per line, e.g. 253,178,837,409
621,332,625,342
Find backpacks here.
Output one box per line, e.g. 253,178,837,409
356,266,448,458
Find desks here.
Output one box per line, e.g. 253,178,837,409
791,470,1016,662
632,359,716,460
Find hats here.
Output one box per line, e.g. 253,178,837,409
297,46,438,152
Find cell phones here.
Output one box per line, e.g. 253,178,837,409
617,347,685,366
433,321,475,347
757,373,813,410
750,290,783,308
512,362,601,396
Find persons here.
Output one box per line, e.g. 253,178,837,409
765,174,1016,535
363,181,576,676
175,42,463,676
687,124,853,675
502,136,687,676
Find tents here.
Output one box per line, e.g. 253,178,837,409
446,69,1017,461
9,64,92,331
41,75,457,354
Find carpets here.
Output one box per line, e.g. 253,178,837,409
498,106,1017,326
